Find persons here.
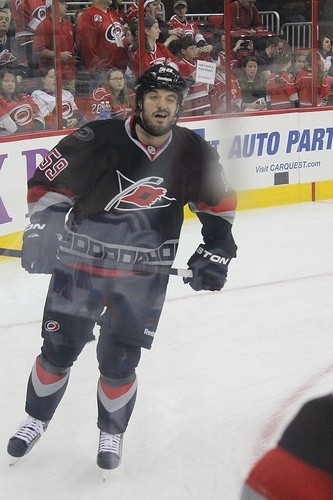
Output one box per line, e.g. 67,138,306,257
7,57,238,481
0,0,332,136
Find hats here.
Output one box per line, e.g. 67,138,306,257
144,0,155,8
173,1,187,7
46,0,52,8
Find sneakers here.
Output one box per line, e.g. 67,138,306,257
97,430,123,482
7,414,49,467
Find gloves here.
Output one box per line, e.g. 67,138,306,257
21,212,65,273
183,243,232,291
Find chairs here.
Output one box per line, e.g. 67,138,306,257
22,15,311,130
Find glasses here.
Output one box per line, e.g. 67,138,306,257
110,76,125,82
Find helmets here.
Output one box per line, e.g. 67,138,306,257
136,65,187,111
